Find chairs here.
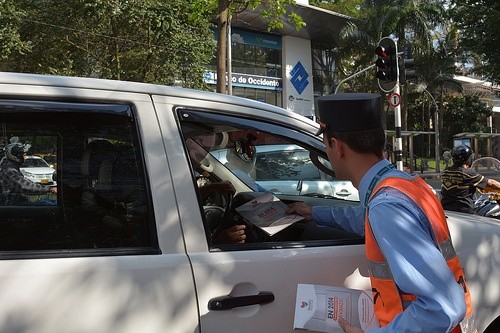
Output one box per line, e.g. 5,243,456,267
78,139,147,248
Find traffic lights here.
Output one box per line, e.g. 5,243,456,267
376,36,399,93
398,51,417,85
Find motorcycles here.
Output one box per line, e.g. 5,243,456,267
471,157,500,219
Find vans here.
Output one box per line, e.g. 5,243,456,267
209,144,359,201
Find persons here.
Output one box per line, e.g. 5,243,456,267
440,145,500,213
0,136,58,206
287,93,473,333
198,181,246,248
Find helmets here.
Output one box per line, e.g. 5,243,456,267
451,145,473,163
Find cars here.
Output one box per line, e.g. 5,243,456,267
18,155,57,184
0,69,499,333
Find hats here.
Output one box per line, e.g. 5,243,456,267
315,93,382,136
